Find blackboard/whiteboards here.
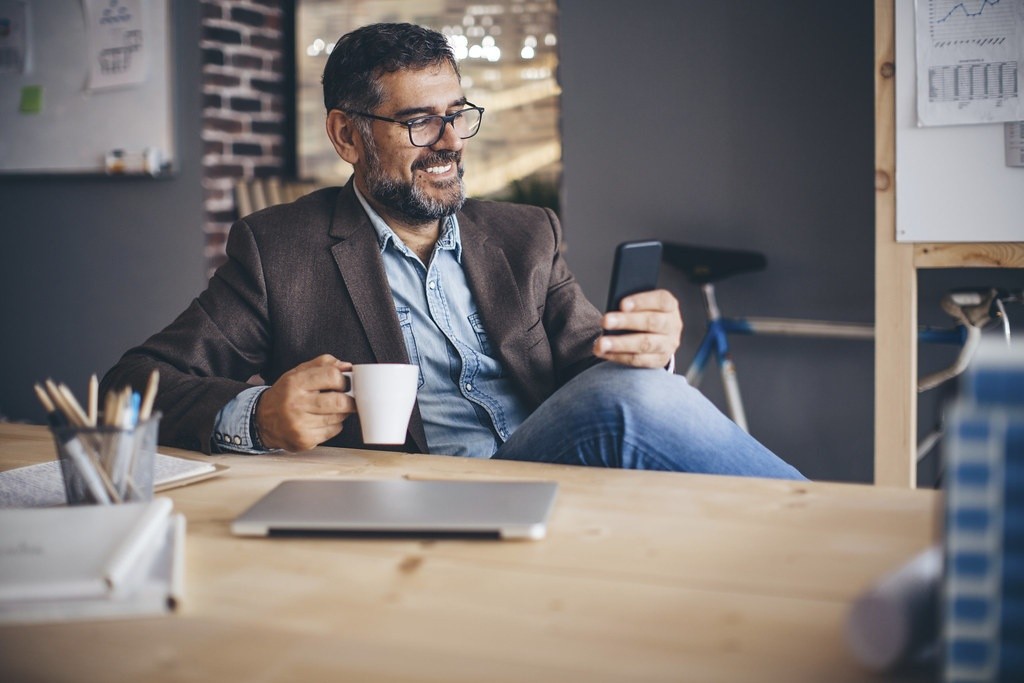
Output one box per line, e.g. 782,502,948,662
0,0,178,175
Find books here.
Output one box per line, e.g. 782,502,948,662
0,496,186,626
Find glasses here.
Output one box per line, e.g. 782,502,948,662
346,100,485,148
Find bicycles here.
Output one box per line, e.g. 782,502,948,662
662,242,1024,463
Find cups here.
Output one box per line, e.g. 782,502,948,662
331,363,419,445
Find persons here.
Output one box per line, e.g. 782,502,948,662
96,22,808,482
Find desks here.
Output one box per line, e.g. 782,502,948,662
1,421,949,682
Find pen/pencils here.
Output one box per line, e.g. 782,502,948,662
33,368,162,505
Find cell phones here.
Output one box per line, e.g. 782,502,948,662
602,241,662,336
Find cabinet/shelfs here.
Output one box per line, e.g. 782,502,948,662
873,0,1024,489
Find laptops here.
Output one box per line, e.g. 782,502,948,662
231,479,558,538
